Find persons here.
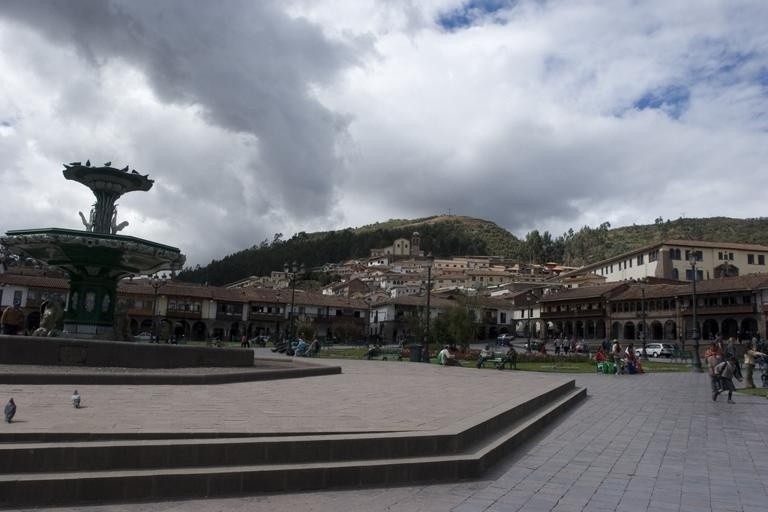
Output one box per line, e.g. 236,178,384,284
259,335,320,357
496,344,515,369
704,329,767,404
594,335,636,375
1,298,25,334
539,335,577,354
150,324,178,345
365,344,379,360
476,346,493,369
437,343,458,366
240,334,250,348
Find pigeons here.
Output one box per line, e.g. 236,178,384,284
70,390,82,411
4,397,17,423
62,158,156,184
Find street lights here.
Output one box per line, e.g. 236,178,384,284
525,291,532,353
638,278,649,359
687,248,703,371
423,248,433,362
146,272,167,341
283,260,306,356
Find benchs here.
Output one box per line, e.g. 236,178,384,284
482,356,516,369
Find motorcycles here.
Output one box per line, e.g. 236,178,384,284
758,355,767,386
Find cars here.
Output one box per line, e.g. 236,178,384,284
571,342,587,352
248,335,268,344
496,332,514,341
524,339,543,351
634,343,672,358
133,332,158,341
